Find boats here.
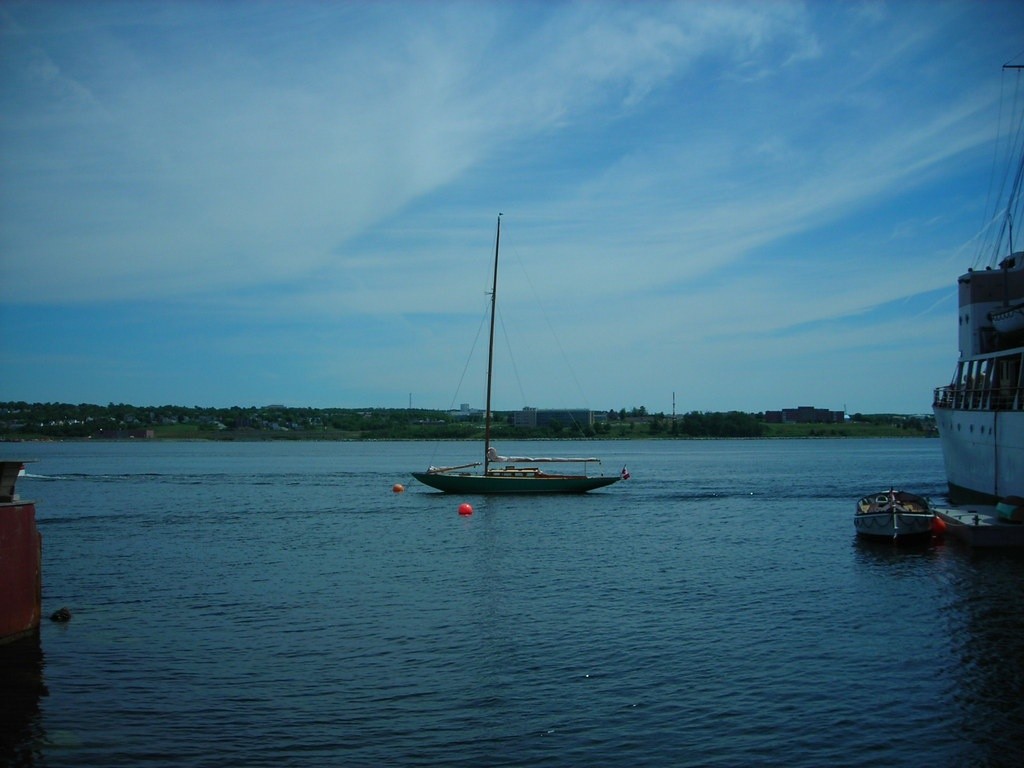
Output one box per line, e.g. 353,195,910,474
854,485,935,541
931,49,1024,506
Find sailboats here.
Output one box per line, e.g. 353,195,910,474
412,214,630,495
